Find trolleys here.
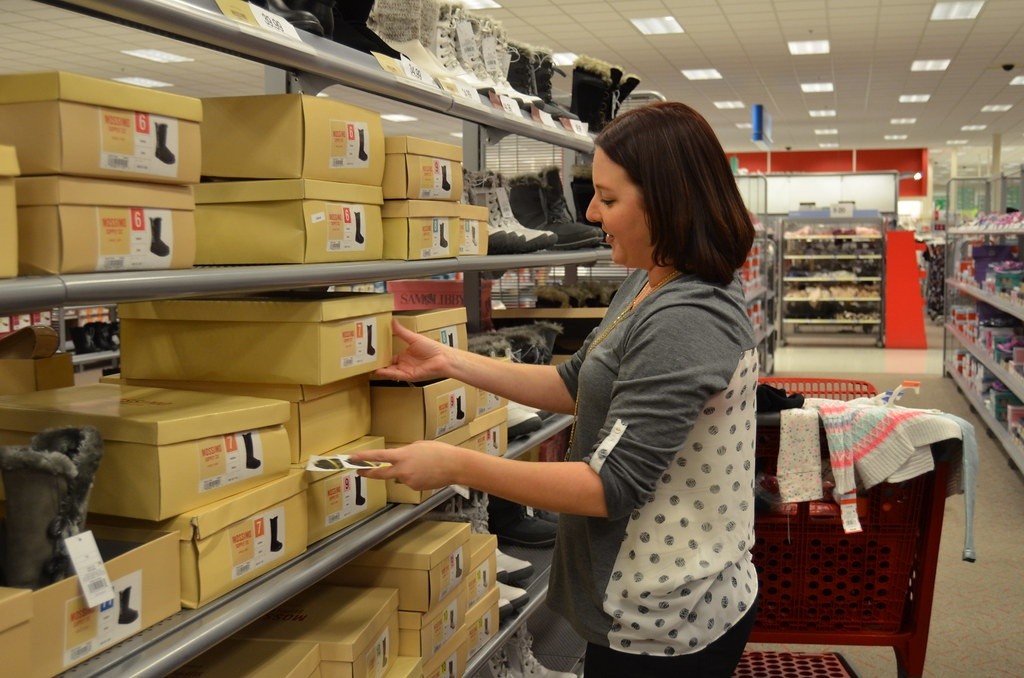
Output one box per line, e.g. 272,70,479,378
693,379,952,677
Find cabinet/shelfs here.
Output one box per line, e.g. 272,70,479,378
778,216,885,348
885,230,929,350
0,0,666,678
942,226,1024,475
745,223,768,378
756,237,775,378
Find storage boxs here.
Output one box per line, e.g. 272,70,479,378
951,211,1024,451
741,240,763,333
0,69,502,678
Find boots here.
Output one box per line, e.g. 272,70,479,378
468,322,564,440
248,0,641,135
449,661,454,678
534,282,619,341
148,217,169,256
485,619,489,635
269,516,283,551
118,586,139,624
355,475,365,505
461,168,611,256
440,165,450,191
382,637,387,667
438,222,448,247
448,333,453,347
471,227,478,246
355,212,364,243
483,571,487,587
455,553,462,577
358,128,367,161
450,610,455,629
456,395,464,419
155,122,176,165
366,324,375,355
242,432,261,469
0,425,103,591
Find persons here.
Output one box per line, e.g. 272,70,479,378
352,102,761,678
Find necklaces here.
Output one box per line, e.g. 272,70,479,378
564,269,679,463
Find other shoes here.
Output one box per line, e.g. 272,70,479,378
478,621,578,678
70,321,121,355
992,381,1008,391
969,211,1024,230
0,324,60,359
997,330,1024,352
426,490,560,619
993,260,1024,275
